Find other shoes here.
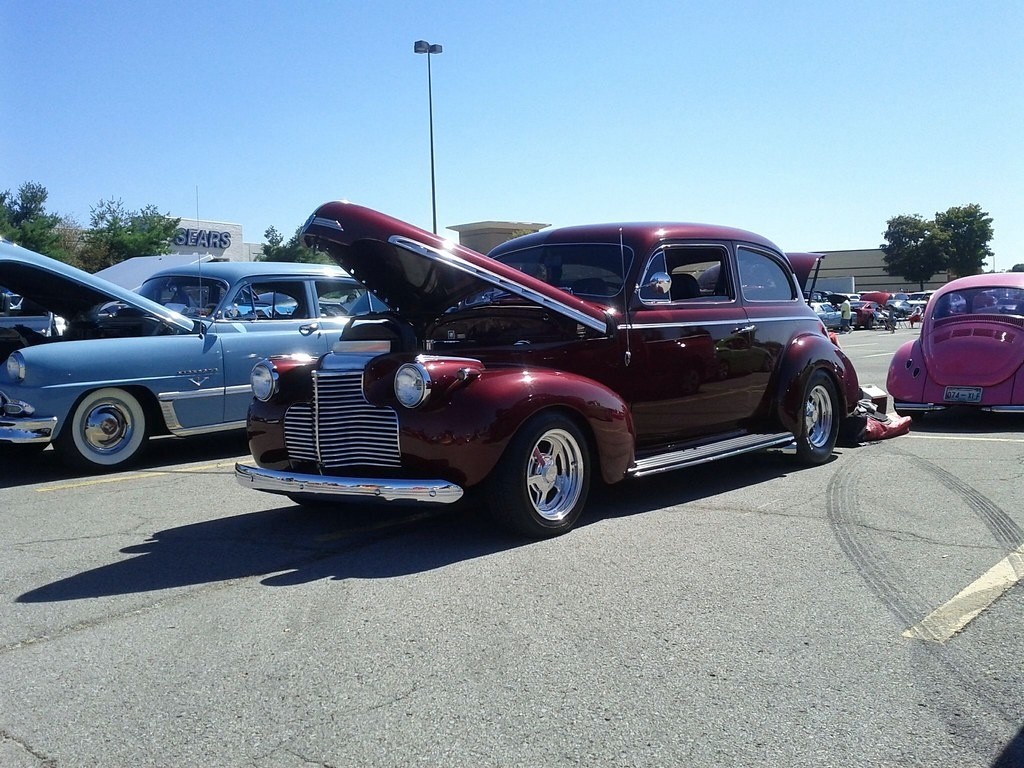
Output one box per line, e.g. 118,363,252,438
848,328,853,334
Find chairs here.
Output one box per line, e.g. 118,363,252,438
872,311,922,331
667,275,700,300
571,278,609,296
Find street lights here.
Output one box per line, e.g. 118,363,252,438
414,40,442,234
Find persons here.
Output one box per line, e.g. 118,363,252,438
909,304,922,328
876,304,898,330
925,296,929,301
901,299,909,306
836,296,853,334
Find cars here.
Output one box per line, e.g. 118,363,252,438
234,201,864,535
0,240,390,476
886,272,1024,426
801,290,1009,332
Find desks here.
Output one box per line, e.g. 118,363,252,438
897,319,909,329
